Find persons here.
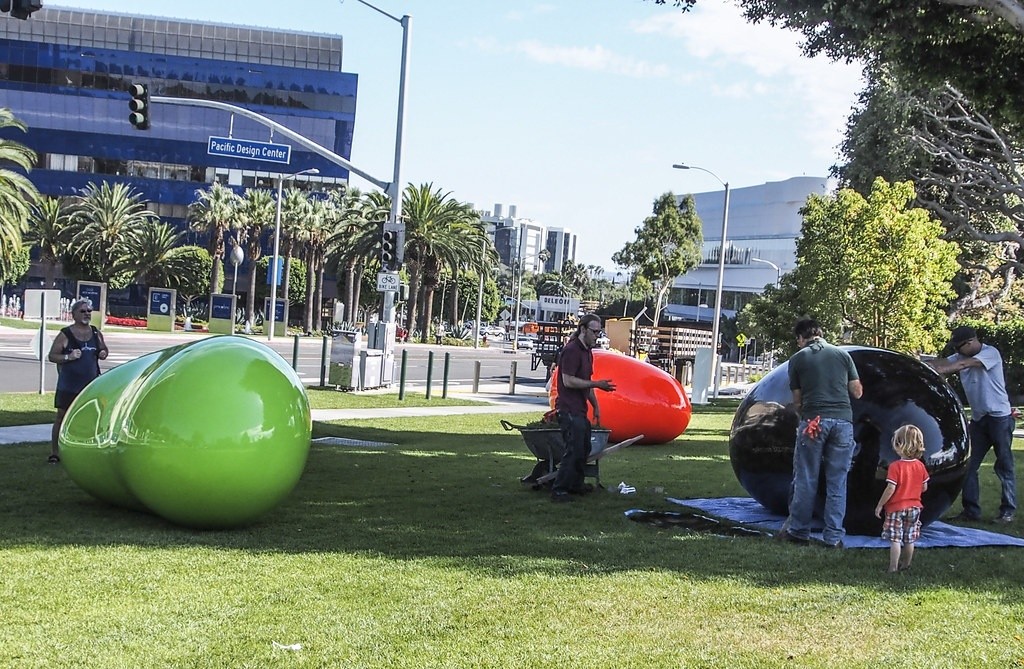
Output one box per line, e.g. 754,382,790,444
47,299,109,462
435,322,444,345
778,318,862,549
875,425,931,573
552,312,617,501
922,327,1017,521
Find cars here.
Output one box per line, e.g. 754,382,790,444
741,354,776,366
517,336,534,350
465,319,506,338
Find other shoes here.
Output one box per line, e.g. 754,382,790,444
947,511,981,523
825,543,837,549
777,530,810,546
551,489,571,502
992,514,1013,525
47,455,59,465
574,482,593,495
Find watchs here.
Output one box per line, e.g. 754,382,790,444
64,354,69,361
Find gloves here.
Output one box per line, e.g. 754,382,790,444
801,415,821,439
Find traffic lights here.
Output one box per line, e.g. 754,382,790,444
129,82,151,130
383,230,398,267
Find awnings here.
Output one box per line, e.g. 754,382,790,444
660,303,724,324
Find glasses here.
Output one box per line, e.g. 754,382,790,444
587,328,603,336
957,339,973,352
795,338,801,349
80,308,92,313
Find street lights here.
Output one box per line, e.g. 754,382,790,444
267,168,320,339
672,164,731,390
514,253,546,351
751,258,782,366
474,226,516,348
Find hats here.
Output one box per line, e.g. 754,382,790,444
949,326,977,343
